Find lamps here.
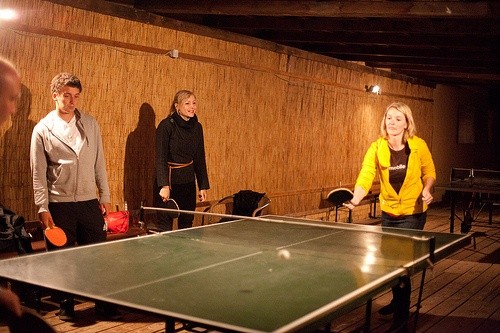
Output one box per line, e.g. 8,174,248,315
372,85,381,95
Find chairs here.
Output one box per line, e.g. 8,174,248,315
208,193,271,224
0,204,146,259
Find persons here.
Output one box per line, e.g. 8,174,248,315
32,75,122,321
152,90,210,233
343,101,436,325
0,58,22,321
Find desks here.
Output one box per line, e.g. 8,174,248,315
0,214,471,333
434,178,500,233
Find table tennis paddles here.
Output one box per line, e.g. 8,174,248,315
44,221,68,247
7,307,58,333
326,187,354,206
165,198,180,219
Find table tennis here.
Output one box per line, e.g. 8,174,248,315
277,249,291,262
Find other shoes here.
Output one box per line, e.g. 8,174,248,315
59,300,75,320
392,304,409,323
378,299,394,315
95,307,124,321
